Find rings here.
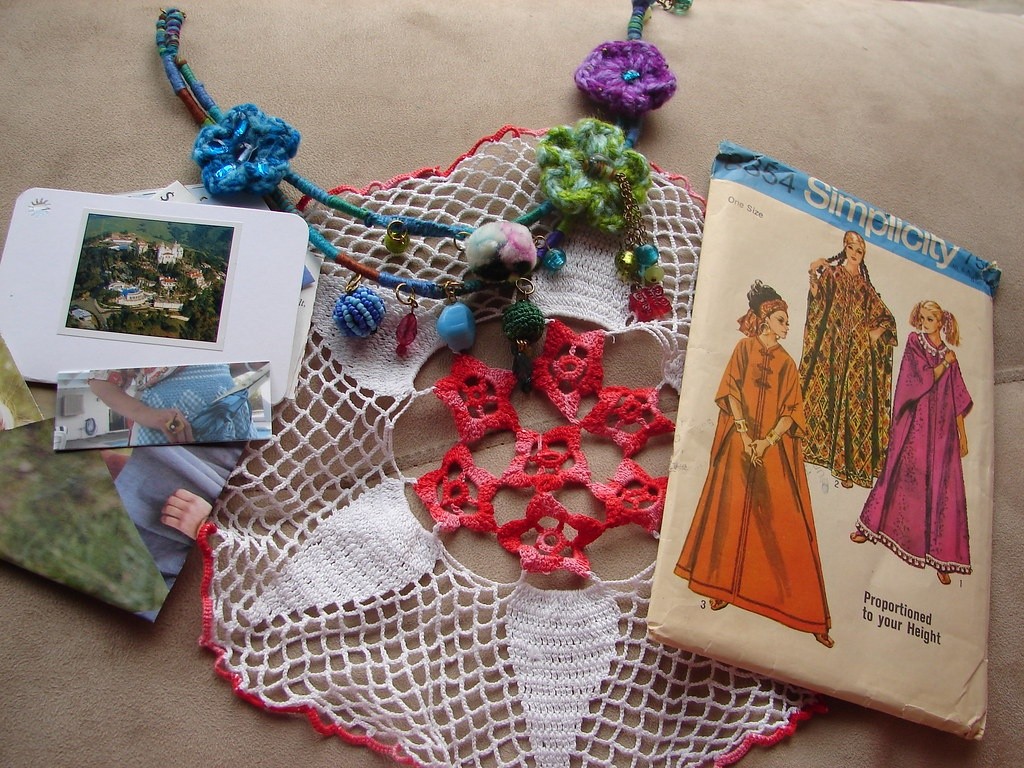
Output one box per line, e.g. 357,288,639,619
166,418,185,434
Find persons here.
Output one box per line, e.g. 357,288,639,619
87,364,261,541
